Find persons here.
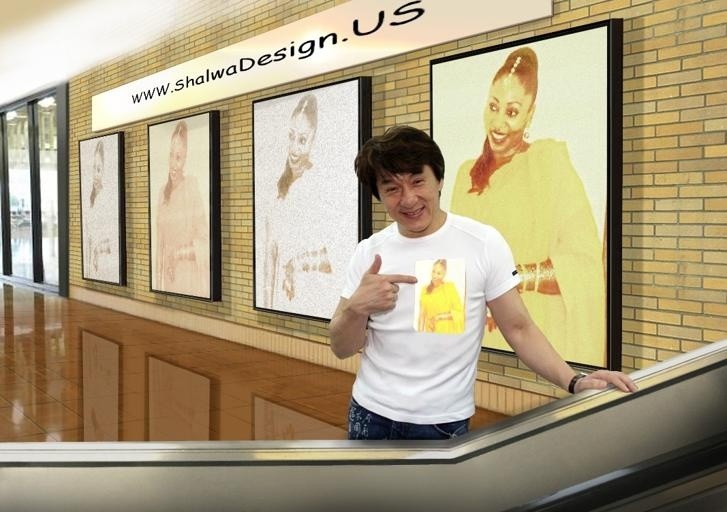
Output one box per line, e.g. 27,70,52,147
449,49,608,368
327,123,636,439
153,121,210,299
84,140,119,281
263,94,342,319
418,259,464,333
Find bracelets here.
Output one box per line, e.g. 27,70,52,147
569,373,587,393
